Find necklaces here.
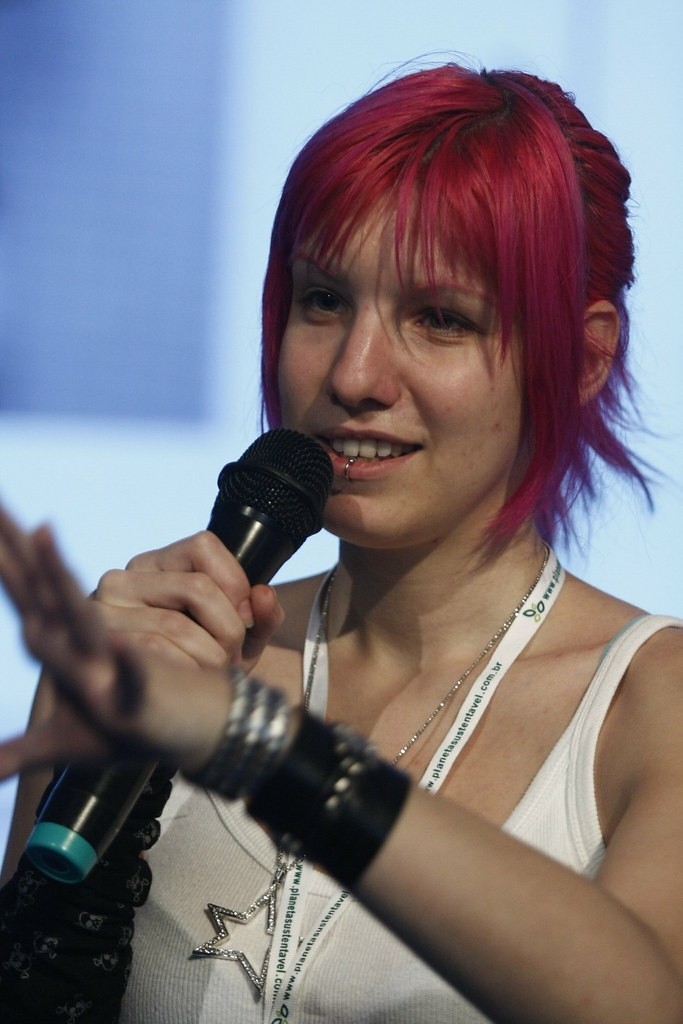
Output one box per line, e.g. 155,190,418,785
192,543,548,996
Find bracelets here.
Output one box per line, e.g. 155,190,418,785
180,665,375,848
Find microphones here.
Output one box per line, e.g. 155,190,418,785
23,427,336,887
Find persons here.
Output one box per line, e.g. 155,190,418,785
0,50,683,1024
